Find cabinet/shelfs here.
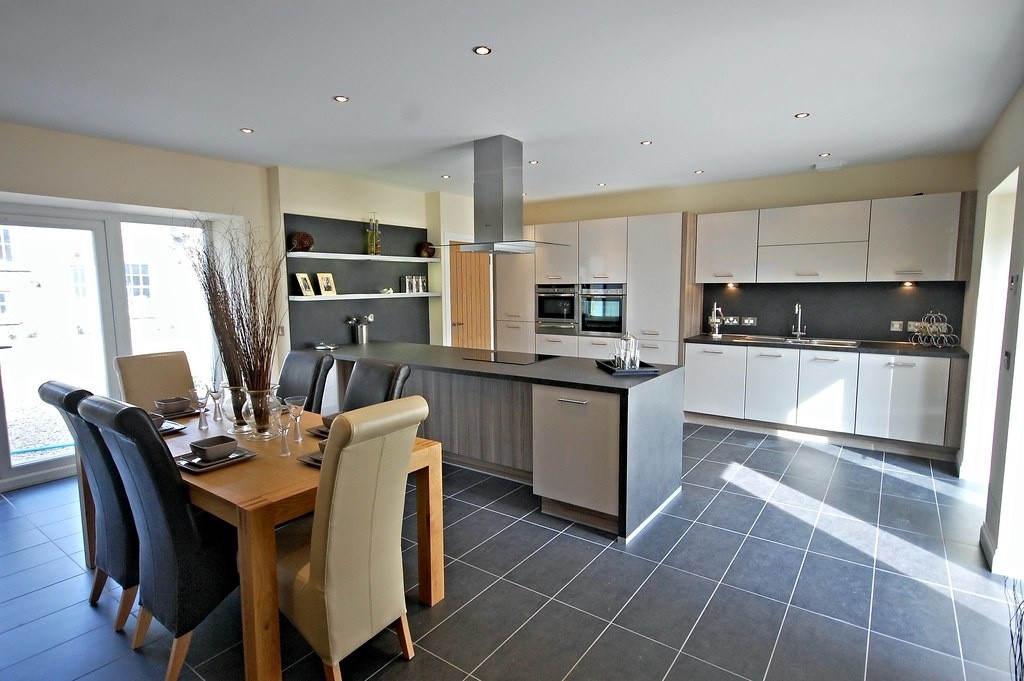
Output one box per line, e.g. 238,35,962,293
694,210,757,284
860,189,961,283
755,197,872,284
744,344,802,432
530,382,621,536
856,352,951,451
679,339,745,421
794,347,860,442
494,210,702,368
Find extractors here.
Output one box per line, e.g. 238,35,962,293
428,133,571,253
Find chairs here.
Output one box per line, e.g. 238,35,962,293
75,393,244,681
37,379,140,632
113,350,201,413
277,350,335,413
274,395,430,680
338,360,412,413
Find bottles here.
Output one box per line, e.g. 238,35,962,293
366,219,381,254
240,382,282,441
221,383,254,434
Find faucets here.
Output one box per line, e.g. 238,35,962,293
791,301,807,339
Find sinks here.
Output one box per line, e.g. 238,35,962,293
732,335,791,345
786,337,862,349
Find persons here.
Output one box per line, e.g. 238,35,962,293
322,277,332,291
303,278,311,291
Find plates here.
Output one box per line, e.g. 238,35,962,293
315,426,329,432
160,420,187,435
180,446,248,467
305,425,329,438
156,408,210,420
296,451,321,467
173,447,259,473
156,407,195,417
308,451,322,462
158,422,174,432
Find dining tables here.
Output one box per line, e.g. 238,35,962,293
157,402,444,681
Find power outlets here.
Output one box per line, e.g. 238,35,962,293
723,316,740,326
742,317,762,326
908,321,929,332
890,320,903,331
934,321,947,333
707,316,722,325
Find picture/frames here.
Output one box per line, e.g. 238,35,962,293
295,273,315,295
316,272,337,296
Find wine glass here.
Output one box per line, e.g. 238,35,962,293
207,383,224,420
188,387,208,430
270,405,295,456
283,395,307,442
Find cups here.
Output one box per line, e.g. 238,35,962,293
608,339,640,369
356,324,369,344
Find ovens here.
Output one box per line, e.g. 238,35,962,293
535,283,627,338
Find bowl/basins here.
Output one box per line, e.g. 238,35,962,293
189,435,238,461
318,439,328,453
322,411,345,429
154,396,190,413
149,412,164,429
